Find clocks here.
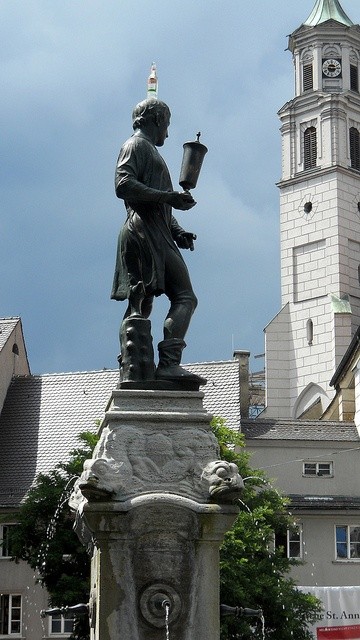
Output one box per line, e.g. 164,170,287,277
322,58,342,78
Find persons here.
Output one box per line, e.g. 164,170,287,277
110,97,207,385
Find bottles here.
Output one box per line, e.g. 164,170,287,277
147,64,159,99
